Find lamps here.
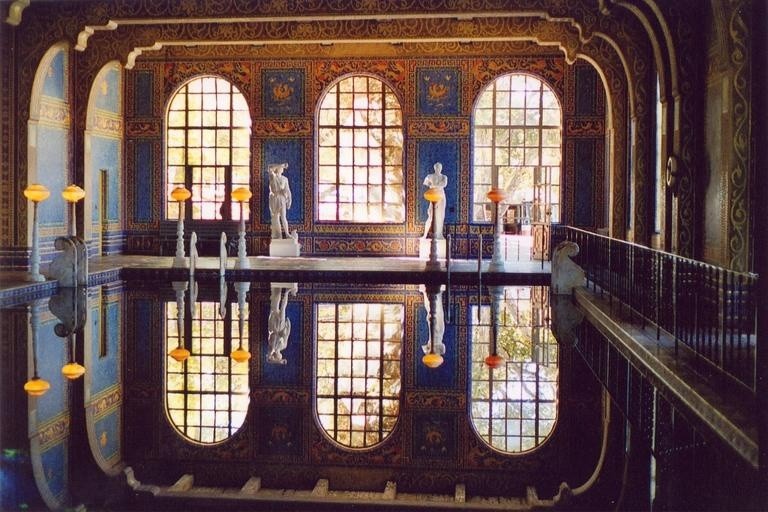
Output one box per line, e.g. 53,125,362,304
230,187,252,270
488,187,503,272
421,281,443,369
170,186,191,268
423,187,442,271
61,334,85,380
485,288,503,368
22,302,50,398
170,281,189,362
230,282,252,363
61,183,86,237
22,183,49,282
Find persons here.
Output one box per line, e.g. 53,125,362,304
418,285,447,354
416,162,448,239
268,162,295,239
265,288,295,365
